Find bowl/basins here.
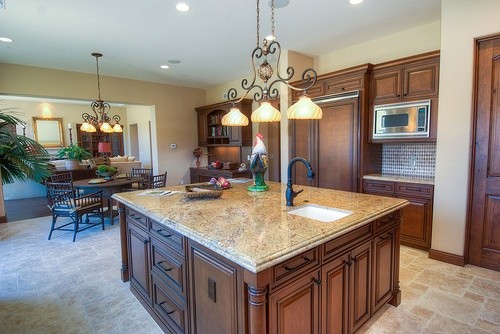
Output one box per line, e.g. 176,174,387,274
128,157,135,160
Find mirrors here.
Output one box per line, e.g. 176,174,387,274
32,118,64,149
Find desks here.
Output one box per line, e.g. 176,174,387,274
73,178,143,226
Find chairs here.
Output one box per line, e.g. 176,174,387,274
45,168,168,242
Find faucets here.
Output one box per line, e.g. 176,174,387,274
285,157,316,206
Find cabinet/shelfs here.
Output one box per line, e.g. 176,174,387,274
368,50,439,104
257,209,401,334
121,199,257,334
76,124,125,158
195,99,253,147
190,168,251,183
363,179,434,252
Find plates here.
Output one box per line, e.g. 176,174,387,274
88,180,107,184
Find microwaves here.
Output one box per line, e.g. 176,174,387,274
371,99,432,138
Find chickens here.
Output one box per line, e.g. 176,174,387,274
249,133,268,187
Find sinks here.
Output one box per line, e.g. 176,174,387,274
282,204,354,222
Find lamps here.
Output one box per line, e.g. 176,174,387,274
80,53,122,133
220,0,323,126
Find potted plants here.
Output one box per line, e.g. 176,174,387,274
57,146,93,170
